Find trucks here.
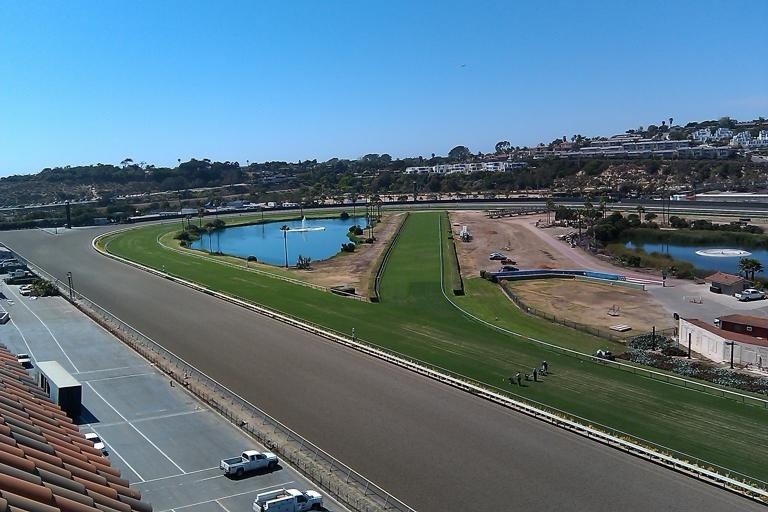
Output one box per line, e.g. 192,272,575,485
252,488,323,512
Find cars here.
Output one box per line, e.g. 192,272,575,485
489,252,520,272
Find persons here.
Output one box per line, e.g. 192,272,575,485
507,360,550,388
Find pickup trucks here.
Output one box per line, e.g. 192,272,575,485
9,269,30,278
735,289,766,302
220,450,279,477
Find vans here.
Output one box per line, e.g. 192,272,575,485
0,259,19,267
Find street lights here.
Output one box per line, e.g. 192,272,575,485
67,271,72,301
